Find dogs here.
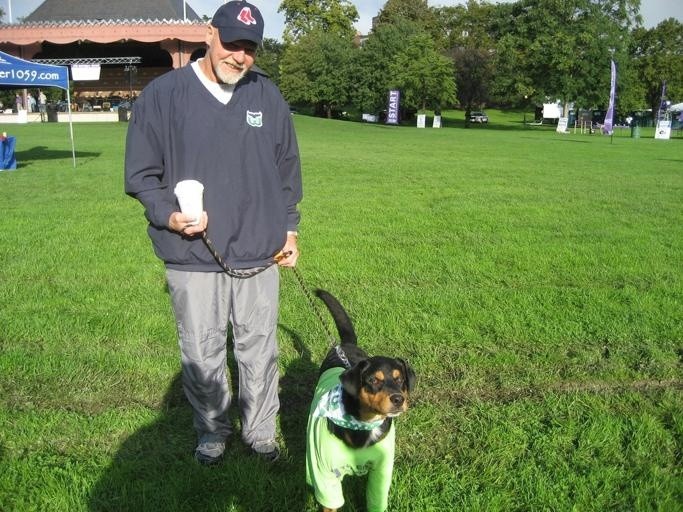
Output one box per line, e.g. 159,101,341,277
305,287,417,512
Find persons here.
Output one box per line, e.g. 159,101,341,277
27,93,35,113
14,93,22,113
122,1,305,466
37,91,48,122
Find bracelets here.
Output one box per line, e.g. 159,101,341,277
286,231,297,236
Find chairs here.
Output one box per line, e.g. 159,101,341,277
573,120,592,134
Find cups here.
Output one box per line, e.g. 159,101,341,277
173,179,205,228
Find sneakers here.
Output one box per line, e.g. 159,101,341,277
251,440,288,466
195,442,226,465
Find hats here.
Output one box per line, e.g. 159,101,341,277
212,0,264,46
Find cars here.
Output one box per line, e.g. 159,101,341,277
329,102,417,123
463,110,488,128
